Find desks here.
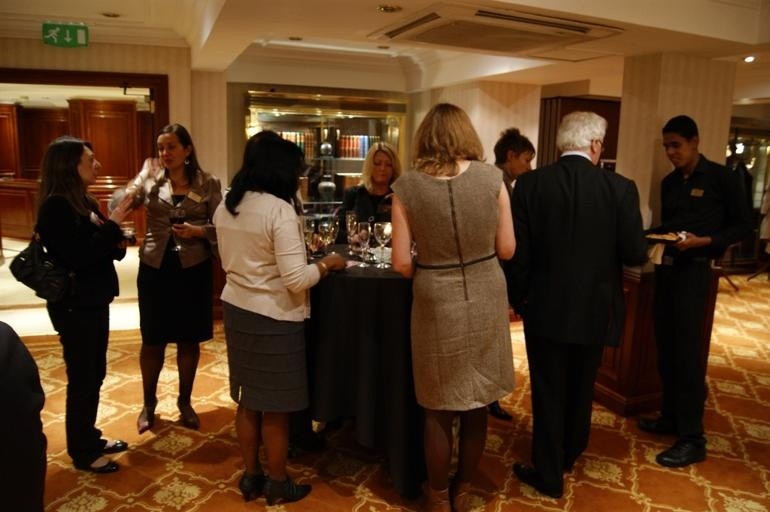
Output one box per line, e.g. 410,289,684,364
291,244,426,500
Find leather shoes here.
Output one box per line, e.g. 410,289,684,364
76,458,119,473
637,416,672,435
104,440,127,454
137,398,158,432
177,395,200,428
512,462,563,498
489,406,512,420
657,439,706,467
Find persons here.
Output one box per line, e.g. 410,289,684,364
503,111,652,498
209,139,347,507
391,102,517,512
0,322,47,511
638,115,743,468
334,141,402,244
32,136,128,473
487,127,535,422
226,130,298,458
107,122,224,435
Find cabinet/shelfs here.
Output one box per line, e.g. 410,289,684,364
592,253,722,417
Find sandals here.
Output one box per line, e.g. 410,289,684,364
421,482,472,512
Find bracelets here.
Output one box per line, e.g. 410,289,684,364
318,260,327,278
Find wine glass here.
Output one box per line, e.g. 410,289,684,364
168,209,187,252
303,210,392,269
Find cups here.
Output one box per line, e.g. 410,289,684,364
121,221,135,238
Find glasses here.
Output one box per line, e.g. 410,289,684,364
590,138,606,152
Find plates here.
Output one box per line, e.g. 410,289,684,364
644,229,680,243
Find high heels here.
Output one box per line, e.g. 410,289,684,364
264,476,312,506
238,472,269,502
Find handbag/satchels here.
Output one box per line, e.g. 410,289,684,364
10,239,77,301
